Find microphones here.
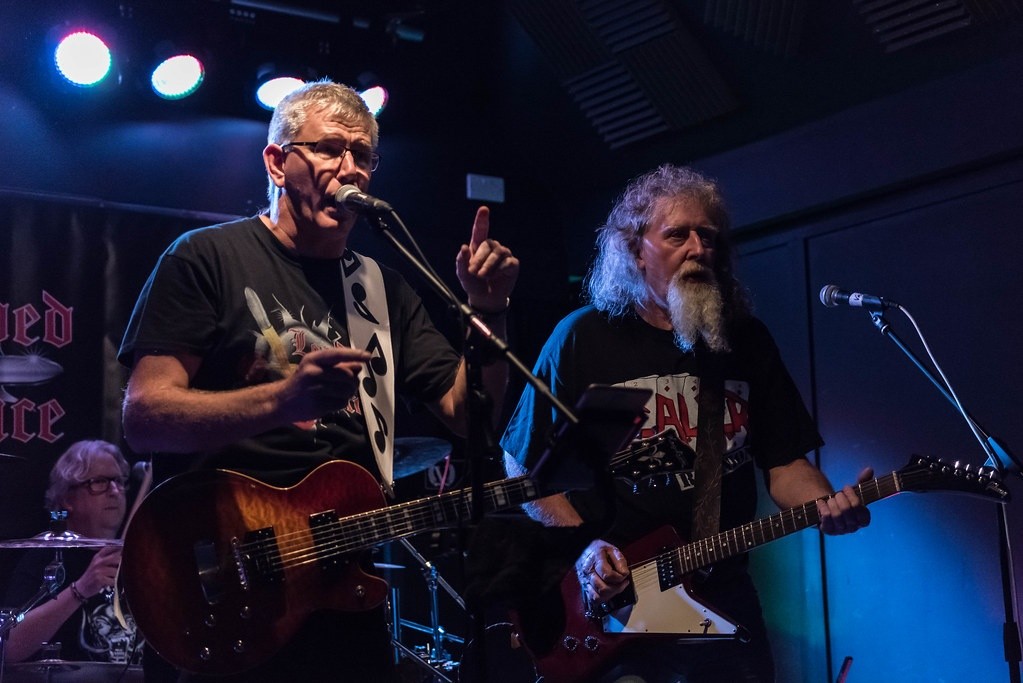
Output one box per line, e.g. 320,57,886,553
335,184,393,214
819,285,900,312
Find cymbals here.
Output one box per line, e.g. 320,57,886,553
394,435,452,485
0,530,124,548
0,656,147,683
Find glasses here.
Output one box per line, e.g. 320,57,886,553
65,478,130,493
280,141,380,171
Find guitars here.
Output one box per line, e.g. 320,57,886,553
118,423,704,683
529,449,1023,683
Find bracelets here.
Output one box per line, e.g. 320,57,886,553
466,296,510,320
70,582,88,604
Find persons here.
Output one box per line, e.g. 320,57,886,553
2,440,147,663
124,82,519,683
498,164,874,683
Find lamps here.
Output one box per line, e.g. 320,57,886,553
341,64,392,119
254,59,311,116
